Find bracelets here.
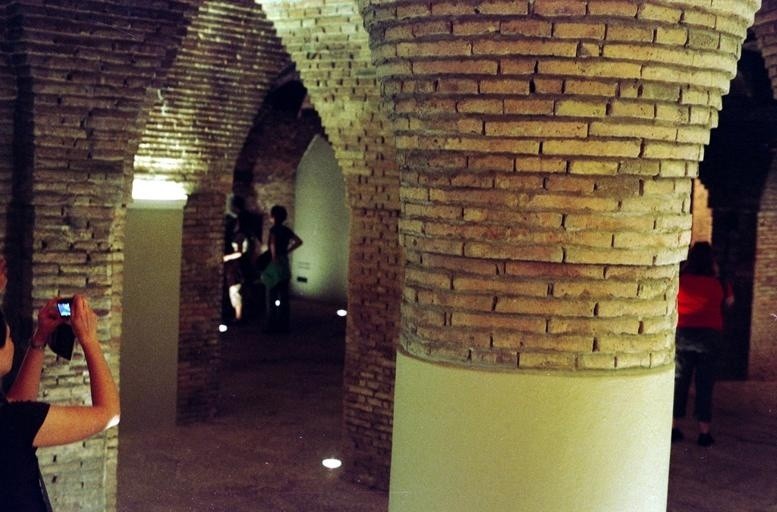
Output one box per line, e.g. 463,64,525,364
28,341,48,350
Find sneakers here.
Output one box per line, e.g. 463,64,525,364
672,428,683,442
698,433,713,446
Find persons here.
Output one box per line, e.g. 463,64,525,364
671,241,737,447
221,186,304,341
1,294,121,511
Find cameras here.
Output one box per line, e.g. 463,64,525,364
56,299,74,319
